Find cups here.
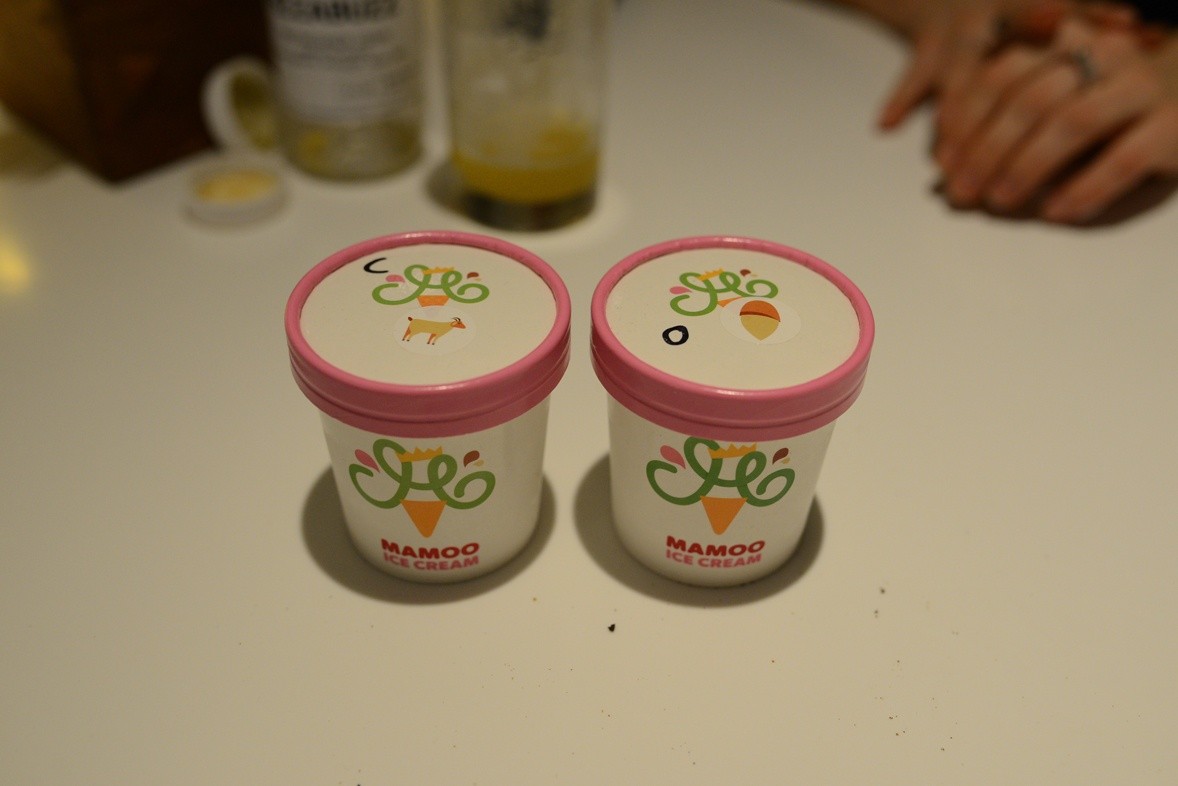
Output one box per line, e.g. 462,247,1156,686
589,236,876,587
433,0,611,225
267,2,432,183
284,232,574,589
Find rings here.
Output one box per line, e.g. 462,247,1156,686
1054,43,1111,87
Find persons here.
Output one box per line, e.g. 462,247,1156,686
848,0,1178,230
